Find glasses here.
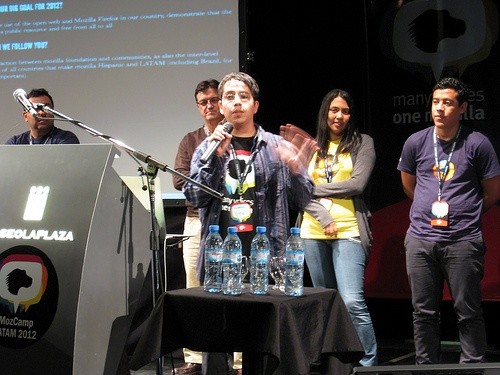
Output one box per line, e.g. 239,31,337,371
223,92,250,102
198,97,219,106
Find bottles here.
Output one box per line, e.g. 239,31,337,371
249,226,271,294
284,228,304,297
222,227,243,295
203,225,224,293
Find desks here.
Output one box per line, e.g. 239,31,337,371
103,283,365,375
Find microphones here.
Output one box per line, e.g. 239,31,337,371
12,88,39,118
200,122,234,164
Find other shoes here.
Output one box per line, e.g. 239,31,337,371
235,368,242,375
171,362,203,375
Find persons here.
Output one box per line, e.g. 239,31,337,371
396,79,500,368
6,88,79,145
172,79,243,375
297,89,379,368
181,72,321,375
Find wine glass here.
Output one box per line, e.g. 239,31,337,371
270,256,284,290
241,255,247,290
